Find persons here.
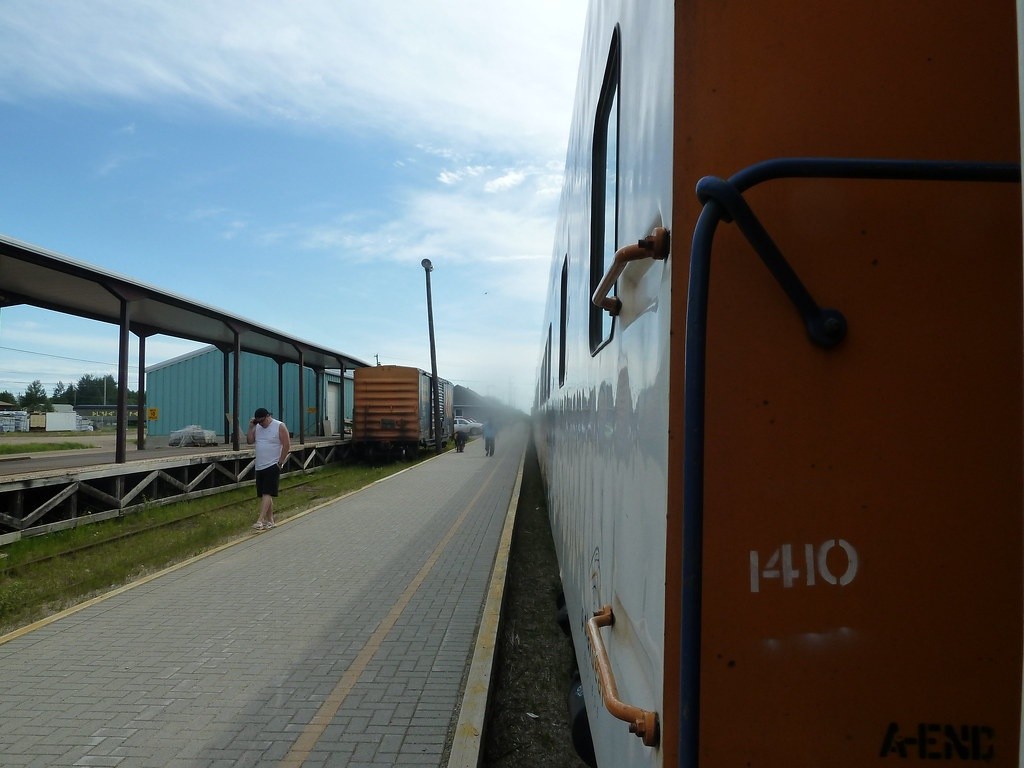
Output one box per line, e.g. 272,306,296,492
247,409,290,530
483,417,498,457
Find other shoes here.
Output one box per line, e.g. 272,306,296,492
252,522,264,530
266,521,277,529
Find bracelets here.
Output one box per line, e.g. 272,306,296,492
278,461,282,467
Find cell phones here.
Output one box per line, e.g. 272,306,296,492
253,419,258,425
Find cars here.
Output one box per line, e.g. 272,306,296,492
458,417,479,423
453,418,483,436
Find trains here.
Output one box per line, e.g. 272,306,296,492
529,0,1023,768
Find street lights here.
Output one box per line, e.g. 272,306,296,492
422,257,443,455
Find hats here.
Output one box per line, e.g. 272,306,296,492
252,408,273,425
